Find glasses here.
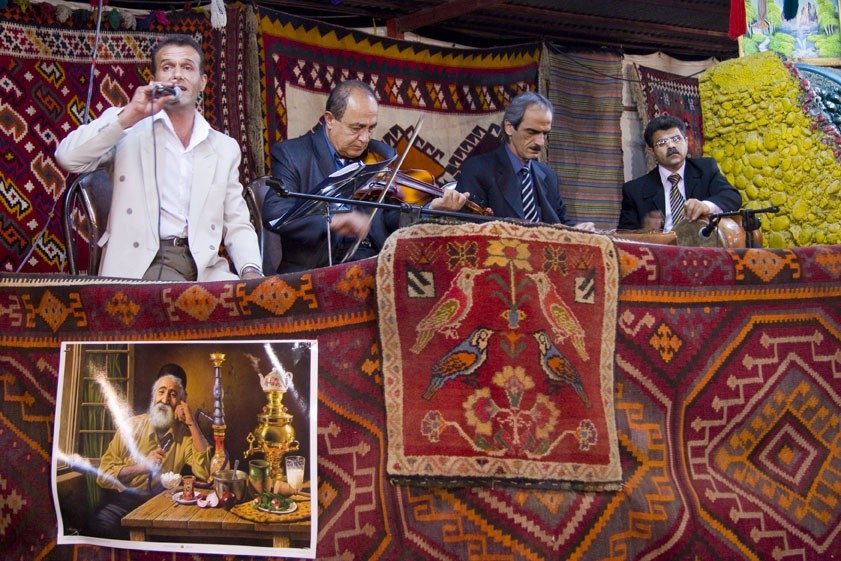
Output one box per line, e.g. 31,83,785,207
653,135,684,149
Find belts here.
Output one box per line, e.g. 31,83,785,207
159,237,188,247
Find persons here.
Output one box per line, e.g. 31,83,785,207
53,36,266,283
457,90,597,232
264,79,472,272
614,114,743,233
86,363,214,539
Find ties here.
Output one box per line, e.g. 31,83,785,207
521,165,540,222
667,173,685,227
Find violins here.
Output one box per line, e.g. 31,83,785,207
352,152,495,218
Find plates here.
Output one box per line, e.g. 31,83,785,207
254,498,297,513
172,491,203,504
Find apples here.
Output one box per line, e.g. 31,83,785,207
220,492,236,511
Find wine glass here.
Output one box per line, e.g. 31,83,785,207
160,475,182,498
285,456,305,501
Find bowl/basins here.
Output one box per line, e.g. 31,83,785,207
212,470,247,504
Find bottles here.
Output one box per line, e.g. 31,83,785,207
182,475,196,500
249,459,271,494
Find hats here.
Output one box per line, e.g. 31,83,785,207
158,364,187,390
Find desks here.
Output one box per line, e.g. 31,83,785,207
121,483,311,549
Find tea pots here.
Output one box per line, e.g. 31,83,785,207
258,367,293,392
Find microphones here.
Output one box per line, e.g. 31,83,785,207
154,82,181,104
698,222,716,240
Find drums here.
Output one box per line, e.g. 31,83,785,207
668,210,765,250
